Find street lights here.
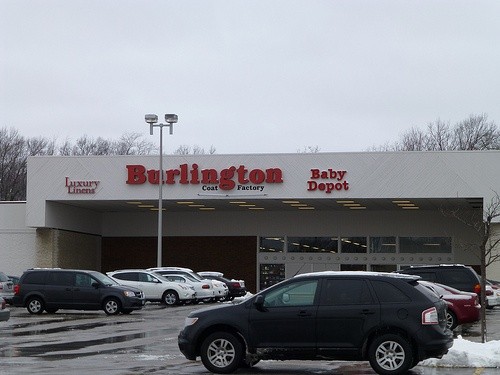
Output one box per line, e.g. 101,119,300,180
145,114,178,267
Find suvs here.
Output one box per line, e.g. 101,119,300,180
177,271,454,375
13,268,147,315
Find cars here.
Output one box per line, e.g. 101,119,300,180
149,266,247,305
106,269,197,307
391,265,500,324
0,271,19,309
